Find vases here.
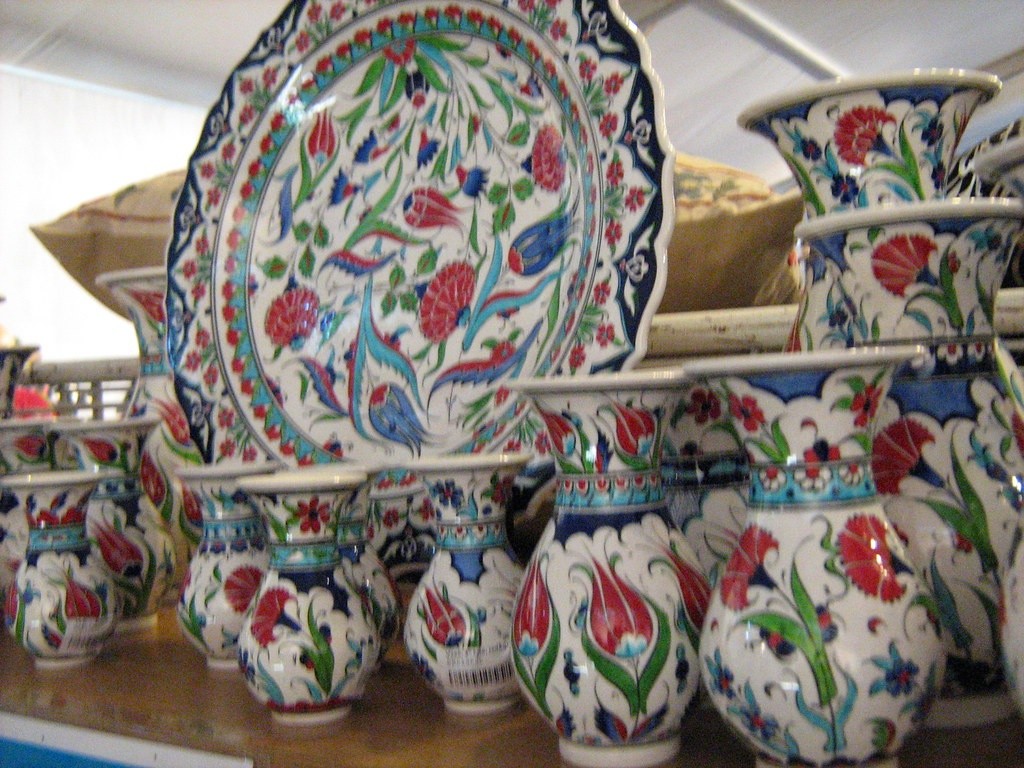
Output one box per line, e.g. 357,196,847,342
792,197,1024,730
0,420,81,641
280,464,404,668
0,346,41,420
96,263,204,612
507,361,710,768
174,461,275,671
407,453,533,717
0,468,120,672
55,417,179,638
235,473,381,730
734,67,1002,219
687,341,947,768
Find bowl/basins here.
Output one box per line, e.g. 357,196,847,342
28,164,805,315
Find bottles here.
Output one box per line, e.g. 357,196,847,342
0,70,1024,768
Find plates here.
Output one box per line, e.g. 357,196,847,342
162,0,677,592
